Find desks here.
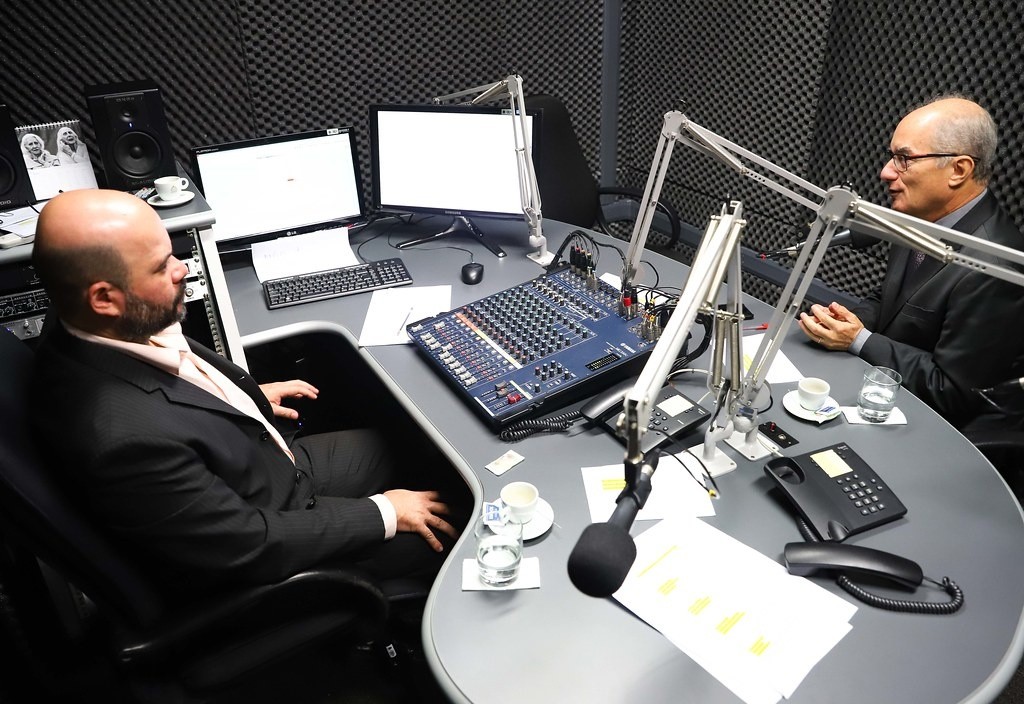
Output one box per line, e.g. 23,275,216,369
224,217,1024,704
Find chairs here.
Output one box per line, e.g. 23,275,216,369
506,93,691,268
0,324,430,704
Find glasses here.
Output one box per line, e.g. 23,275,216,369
888,148,982,172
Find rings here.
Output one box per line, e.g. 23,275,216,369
818,338,821,344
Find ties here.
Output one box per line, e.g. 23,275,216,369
900,249,925,293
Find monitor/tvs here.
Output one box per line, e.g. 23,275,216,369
368,103,542,258
191,126,369,256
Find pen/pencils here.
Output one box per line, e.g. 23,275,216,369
743,324,768,330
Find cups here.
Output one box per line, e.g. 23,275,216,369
154,176,189,201
856,365,902,424
500,482,539,525
474,510,524,586
797,377,831,411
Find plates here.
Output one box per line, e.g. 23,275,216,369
147,190,195,207
486,498,554,540
782,390,840,422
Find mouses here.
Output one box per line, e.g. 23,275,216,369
461,262,483,285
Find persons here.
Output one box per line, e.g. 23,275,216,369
32,189,461,684
56,127,91,165
798,90,1024,499
20,134,61,170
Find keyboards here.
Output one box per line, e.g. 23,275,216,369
262,258,414,309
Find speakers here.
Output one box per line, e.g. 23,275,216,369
84,79,180,191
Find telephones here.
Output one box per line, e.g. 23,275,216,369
764,442,922,594
579,375,711,454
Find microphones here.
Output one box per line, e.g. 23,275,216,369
567,447,662,598
757,228,883,260
